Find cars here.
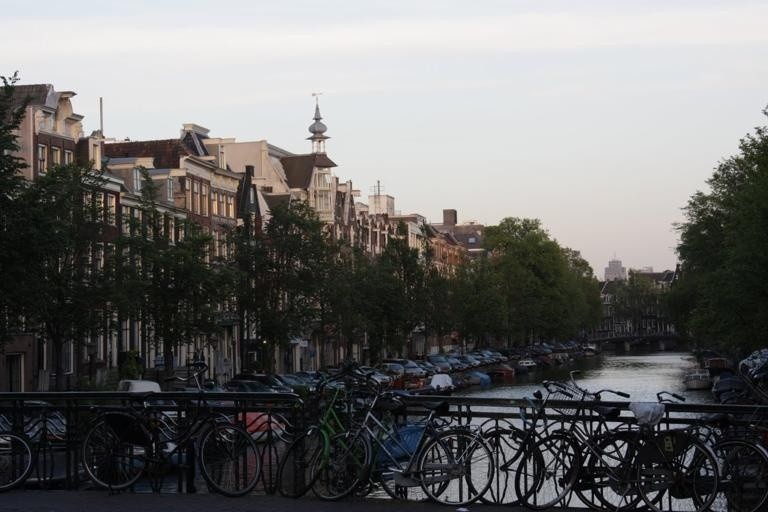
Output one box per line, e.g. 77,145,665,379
476,338,582,366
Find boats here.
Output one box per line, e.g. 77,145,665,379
582,342,602,351
